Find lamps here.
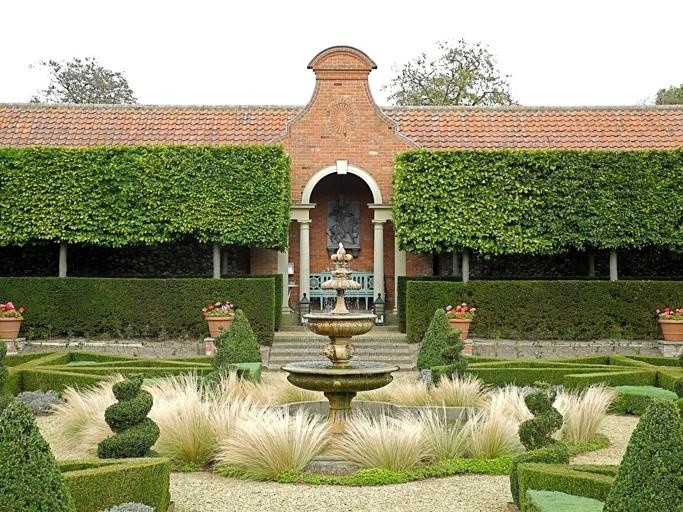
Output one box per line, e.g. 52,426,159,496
299,293,311,326
372,293,386,326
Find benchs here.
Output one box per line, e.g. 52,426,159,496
310,271,374,312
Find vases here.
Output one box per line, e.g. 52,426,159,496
0,316,24,339
204,315,234,338
658,319,683,341
448,319,472,340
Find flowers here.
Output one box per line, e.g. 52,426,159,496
655,307,683,320
445,302,477,320
0,301,26,318
202,301,234,317
